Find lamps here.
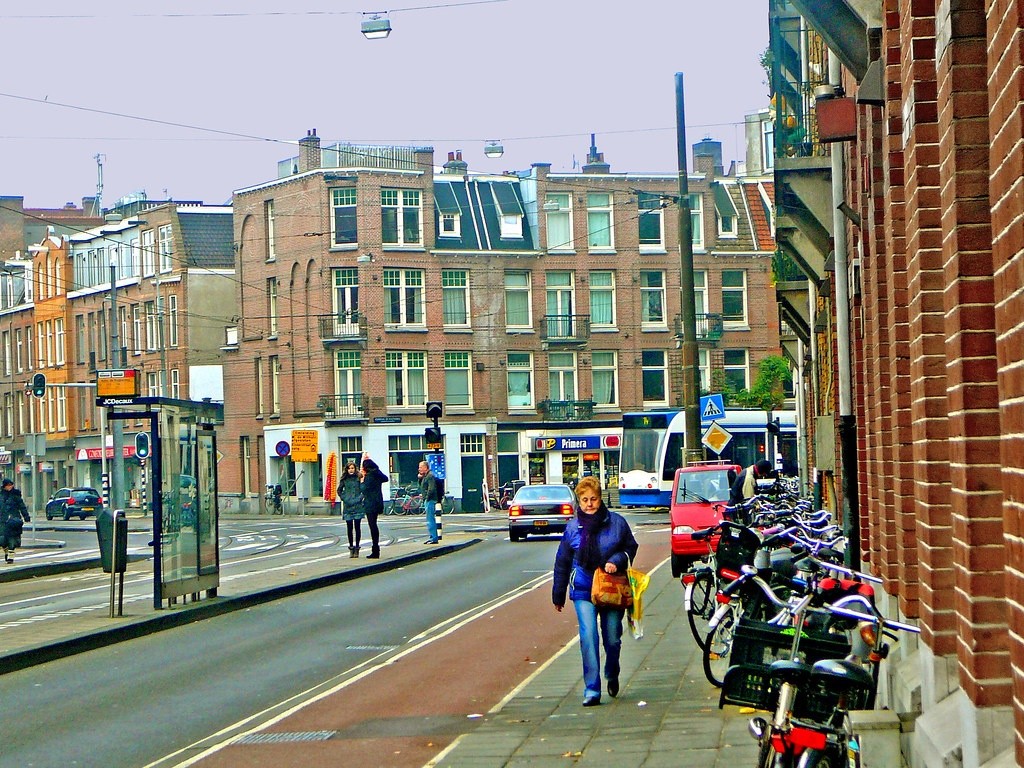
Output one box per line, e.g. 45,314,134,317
484,140,504,158
361,11,393,39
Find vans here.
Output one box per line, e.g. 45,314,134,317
670,459,745,578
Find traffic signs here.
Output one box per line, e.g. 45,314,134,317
427,454,446,479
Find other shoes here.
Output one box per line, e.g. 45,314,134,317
423,540,439,544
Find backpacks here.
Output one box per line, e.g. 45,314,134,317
434,477,444,503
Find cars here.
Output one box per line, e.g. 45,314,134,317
178,474,200,527
508,484,580,541
46,487,103,520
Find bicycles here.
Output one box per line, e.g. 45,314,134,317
384,482,457,516
488,481,514,510
681,476,922,767
263,483,285,515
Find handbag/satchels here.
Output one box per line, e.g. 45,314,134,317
592,564,635,606
623,551,651,639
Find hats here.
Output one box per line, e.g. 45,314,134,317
3,479,13,486
757,460,772,476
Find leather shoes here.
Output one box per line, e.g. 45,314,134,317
582,696,601,706
607,679,619,697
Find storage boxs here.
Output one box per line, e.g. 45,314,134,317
725,617,878,726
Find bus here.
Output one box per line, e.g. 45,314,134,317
619,406,801,506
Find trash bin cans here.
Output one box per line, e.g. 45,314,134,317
96,509,128,573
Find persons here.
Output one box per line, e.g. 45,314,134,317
418,461,438,544
552,477,639,706
0,479,30,564
726,460,772,511
361,459,389,558
337,463,364,558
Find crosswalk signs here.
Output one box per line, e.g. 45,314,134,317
700,394,726,422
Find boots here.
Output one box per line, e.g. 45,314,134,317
348,546,354,558
4,550,10,562
366,546,379,559
7,550,14,563
354,547,360,558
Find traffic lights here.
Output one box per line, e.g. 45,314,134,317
32,373,46,398
425,400,443,418
134,432,150,459
424,427,441,443
767,418,781,435
